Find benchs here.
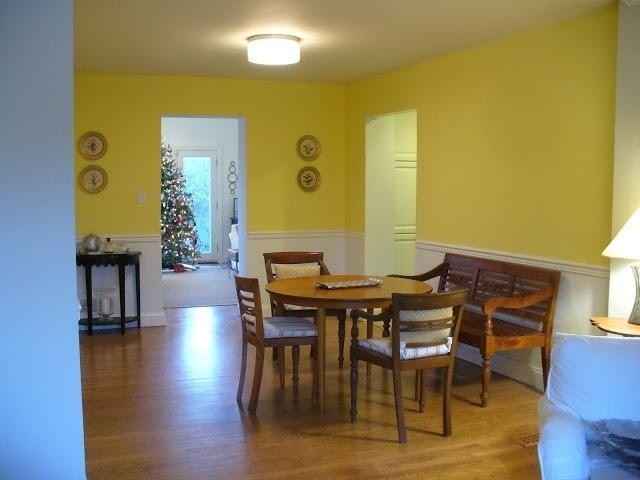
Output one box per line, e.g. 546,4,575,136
400,252,561,408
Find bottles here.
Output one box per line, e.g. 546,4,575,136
104,238,113,253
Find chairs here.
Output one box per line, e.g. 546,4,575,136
261,251,347,368
349,288,469,443
537,331,640,480
235,277,319,411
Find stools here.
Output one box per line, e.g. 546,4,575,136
227,248,238,279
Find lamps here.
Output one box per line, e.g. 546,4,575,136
601,205,640,325
246,34,302,65
79,165,108,194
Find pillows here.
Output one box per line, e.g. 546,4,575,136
274,264,321,281
399,307,452,343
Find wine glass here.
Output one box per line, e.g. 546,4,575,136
95,289,115,322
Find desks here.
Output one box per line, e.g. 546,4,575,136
589,316,640,338
76,249,143,335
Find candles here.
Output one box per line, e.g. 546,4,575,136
97,296,113,322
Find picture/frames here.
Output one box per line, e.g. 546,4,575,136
296,135,321,162
76,131,108,161
297,165,321,192
